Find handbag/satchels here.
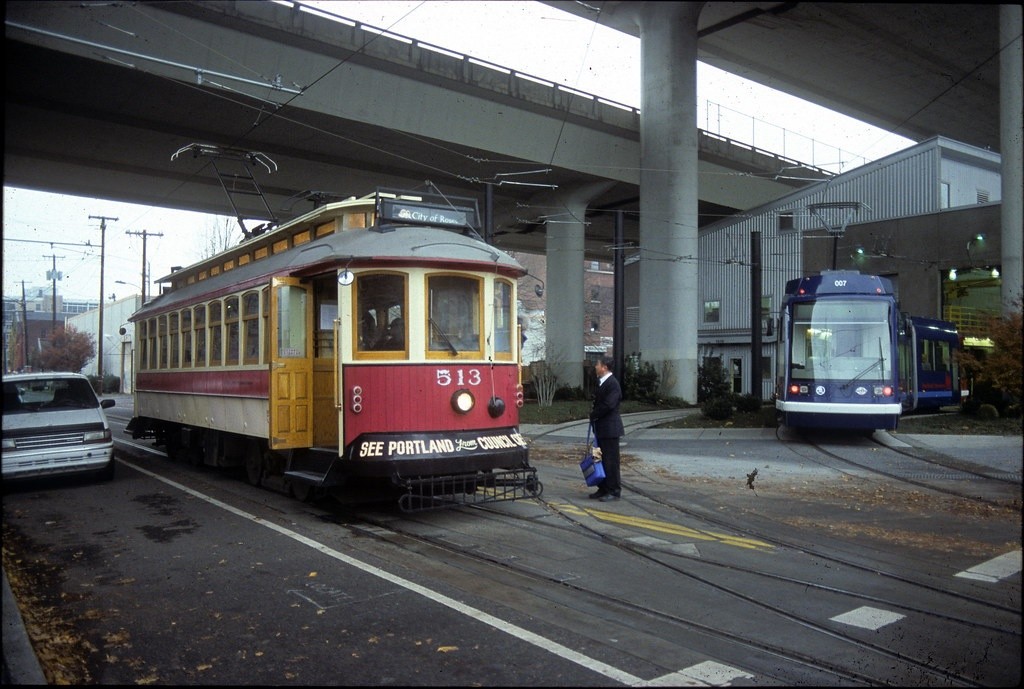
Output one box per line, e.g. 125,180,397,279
581,456,606,486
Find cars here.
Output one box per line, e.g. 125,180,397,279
2,370,120,483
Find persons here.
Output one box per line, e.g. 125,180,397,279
0,384,86,412
141,299,382,374
587,355,626,503
386,317,405,350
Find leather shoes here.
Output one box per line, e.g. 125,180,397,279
591,489,620,501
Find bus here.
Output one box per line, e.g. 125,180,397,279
129,190,546,518
765,269,962,430
